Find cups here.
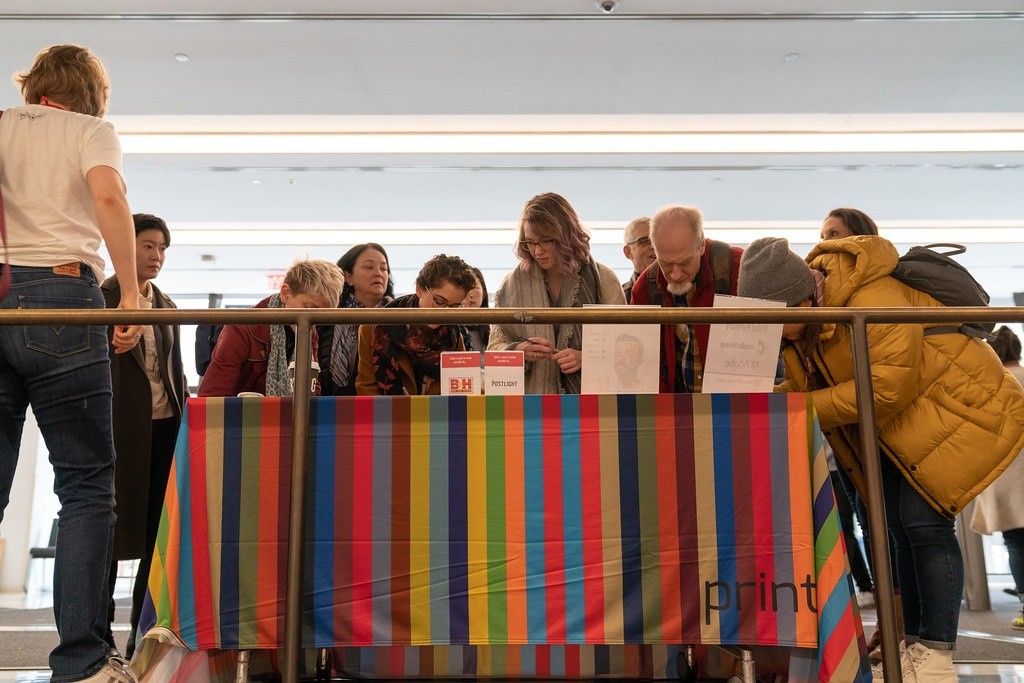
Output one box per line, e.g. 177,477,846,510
288,361,321,396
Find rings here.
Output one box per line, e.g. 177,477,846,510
133,332,141,341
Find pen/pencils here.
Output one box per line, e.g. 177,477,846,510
520,336,537,345
402,387,409,395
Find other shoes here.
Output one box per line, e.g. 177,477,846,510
68,660,134,683
1004,587,1018,595
865,625,888,664
855,590,877,609
1011,615,1024,630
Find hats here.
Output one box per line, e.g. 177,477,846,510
737,238,816,307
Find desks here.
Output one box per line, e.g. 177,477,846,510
128,392,873,683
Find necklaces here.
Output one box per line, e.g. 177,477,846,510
40,96,64,110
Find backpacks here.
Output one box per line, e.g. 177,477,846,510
890,242,996,338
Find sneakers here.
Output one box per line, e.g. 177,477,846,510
869,640,959,683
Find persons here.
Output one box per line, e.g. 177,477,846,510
736,232,1024,683
486,192,628,394
315,242,395,396
198,260,344,397
614,333,644,379
630,207,745,393
355,254,477,394
621,217,656,305
100,212,190,660
820,207,1024,631
0,45,145,683
462,268,491,351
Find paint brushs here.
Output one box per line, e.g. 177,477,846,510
109,327,128,356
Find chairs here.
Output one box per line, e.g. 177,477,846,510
27,518,59,593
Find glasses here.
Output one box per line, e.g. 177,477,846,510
626,235,650,248
424,283,464,308
519,235,558,252
